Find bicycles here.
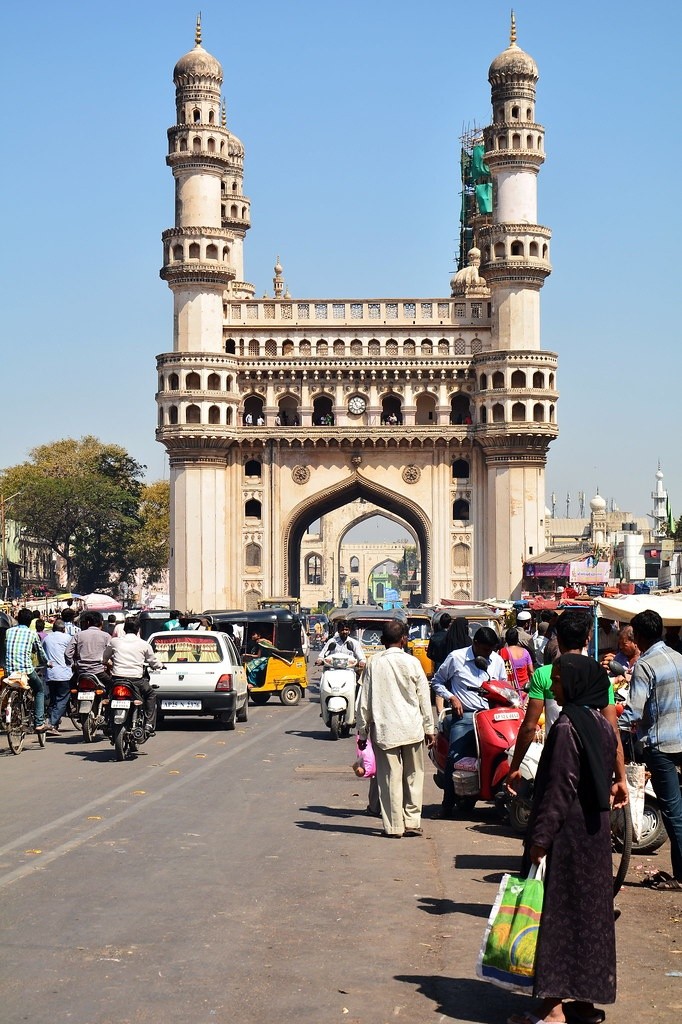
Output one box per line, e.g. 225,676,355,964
4,674,48,755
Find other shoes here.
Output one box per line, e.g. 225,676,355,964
107,720,114,730
381,830,401,839
46,728,62,736
431,809,453,819
405,827,423,836
367,805,380,816
144,725,156,737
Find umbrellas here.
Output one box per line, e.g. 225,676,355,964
53,592,122,611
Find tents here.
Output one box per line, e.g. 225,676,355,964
593,594,682,664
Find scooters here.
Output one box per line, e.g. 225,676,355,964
103,680,146,760
69,671,110,742
426,651,533,829
315,652,360,738
505,734,666,856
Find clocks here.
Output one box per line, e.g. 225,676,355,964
348,396,366,415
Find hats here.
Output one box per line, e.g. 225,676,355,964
517,611,531,620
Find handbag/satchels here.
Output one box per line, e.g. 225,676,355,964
475,854,547,995
356,735,376,778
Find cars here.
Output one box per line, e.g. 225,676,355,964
140,630,250,730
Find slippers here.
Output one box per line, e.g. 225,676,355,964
563,1002,605,1024
651,876,682,892
640,871,672,886
35,724,53,732
507,1011,569,1024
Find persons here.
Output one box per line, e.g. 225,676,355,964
450,413,472,424
314,611,682,891
506,653,618,1024
0,611,271,738
246,410,332,426
381,412,398,425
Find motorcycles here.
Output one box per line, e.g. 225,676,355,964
332,602,508,680
194,608,309,707
